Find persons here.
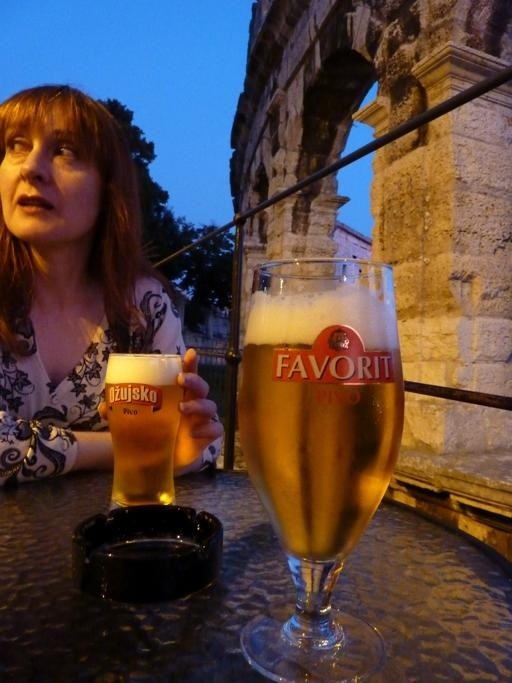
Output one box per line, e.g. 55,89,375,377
0,84,225,489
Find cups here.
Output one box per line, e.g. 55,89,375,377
102,348,188,508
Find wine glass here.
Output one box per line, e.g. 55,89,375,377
239,257,406,683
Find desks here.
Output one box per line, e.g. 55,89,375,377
0,470,512,682
